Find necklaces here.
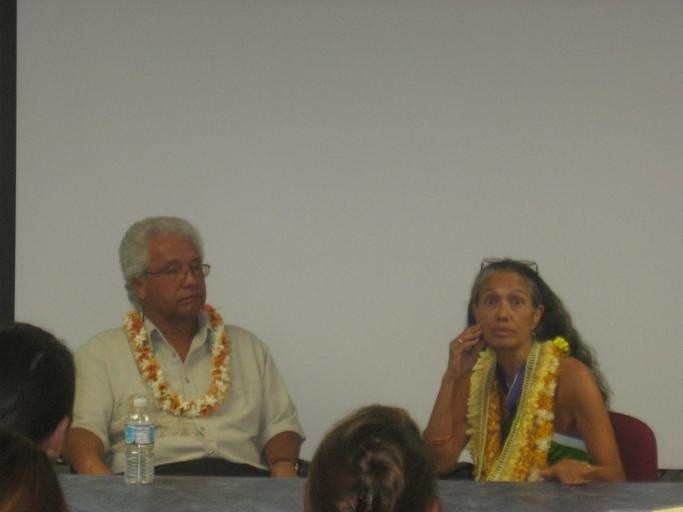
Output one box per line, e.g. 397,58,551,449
123,303,231,418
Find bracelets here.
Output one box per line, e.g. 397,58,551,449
424,430,454,444
268,455,297,472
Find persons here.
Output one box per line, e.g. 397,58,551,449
304,403,442,511
421,257,626,484
60,216,307,477
1,320,75,464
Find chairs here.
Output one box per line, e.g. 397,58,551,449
576,411,656,484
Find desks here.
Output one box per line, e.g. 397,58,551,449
55,474,682,512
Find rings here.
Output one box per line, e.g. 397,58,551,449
457,337,463,344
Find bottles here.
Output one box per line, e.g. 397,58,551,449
126,398,156,485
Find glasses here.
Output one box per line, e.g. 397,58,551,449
141,262,211,285
478,257,537,282
